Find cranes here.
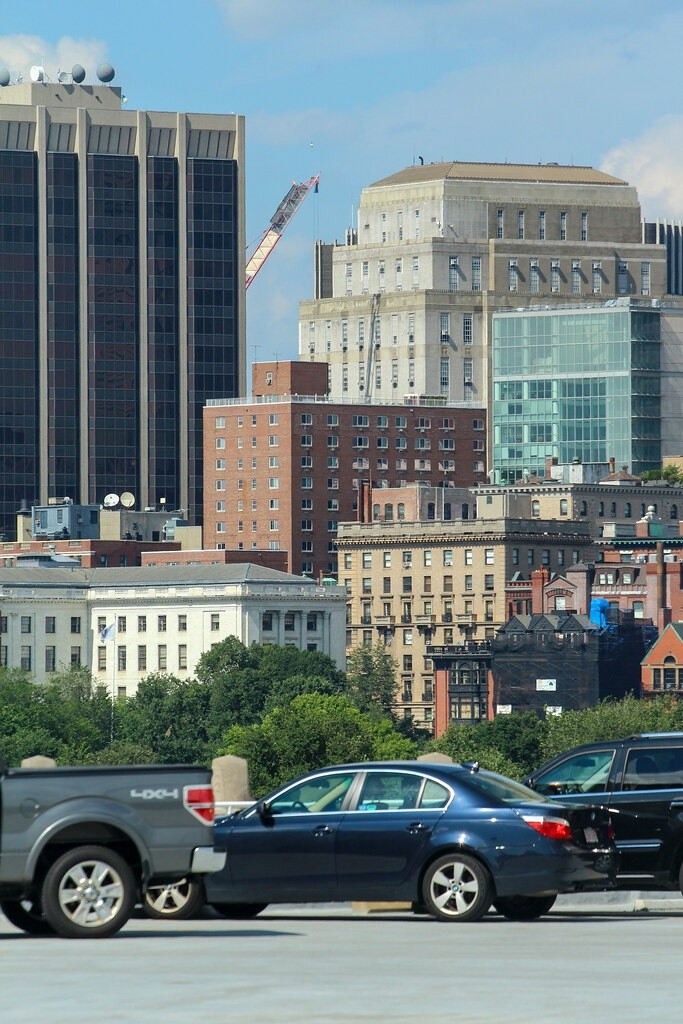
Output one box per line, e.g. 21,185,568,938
245,170,323,289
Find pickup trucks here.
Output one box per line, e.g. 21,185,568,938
0,762,231,941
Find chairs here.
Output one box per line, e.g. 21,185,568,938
364,775,388,809
397,776,424,809
632,756,661,790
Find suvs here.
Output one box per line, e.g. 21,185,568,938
523,732,683,897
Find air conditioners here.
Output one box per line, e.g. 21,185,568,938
551,287,558,293
465,376,472,382
509,286,516,291
441,329,448,335
593,288,601,293
450,257,627,272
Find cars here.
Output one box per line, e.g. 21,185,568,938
139,760,622,922
